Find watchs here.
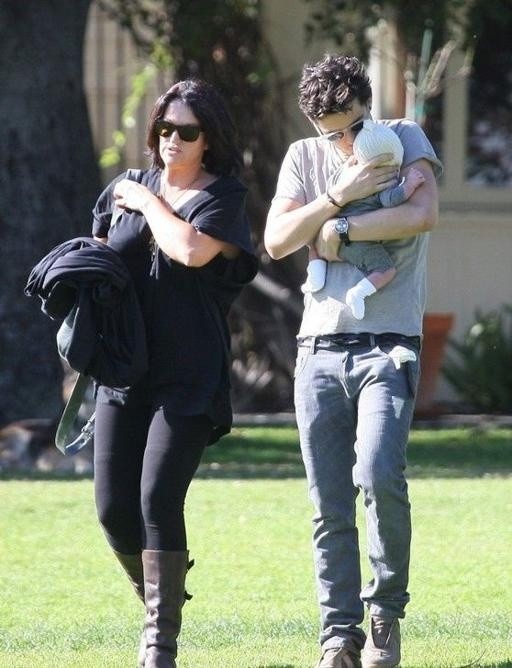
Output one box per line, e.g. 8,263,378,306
334,214,351,247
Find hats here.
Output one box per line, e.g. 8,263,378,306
354,119,404,168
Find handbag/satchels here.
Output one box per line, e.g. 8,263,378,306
54,297,97,386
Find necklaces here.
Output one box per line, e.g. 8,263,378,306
157,172,201,217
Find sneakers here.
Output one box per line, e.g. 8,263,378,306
361,614,401,667
317,647,361,668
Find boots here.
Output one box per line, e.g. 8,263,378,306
111,549,194,668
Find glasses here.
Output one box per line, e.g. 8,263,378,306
154,119,203,142
313,109,368,142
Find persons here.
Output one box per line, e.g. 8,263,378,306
82,74,263,667
289,120,427,324
248,50,449,666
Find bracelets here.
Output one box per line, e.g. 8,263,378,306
326,190,343,209
